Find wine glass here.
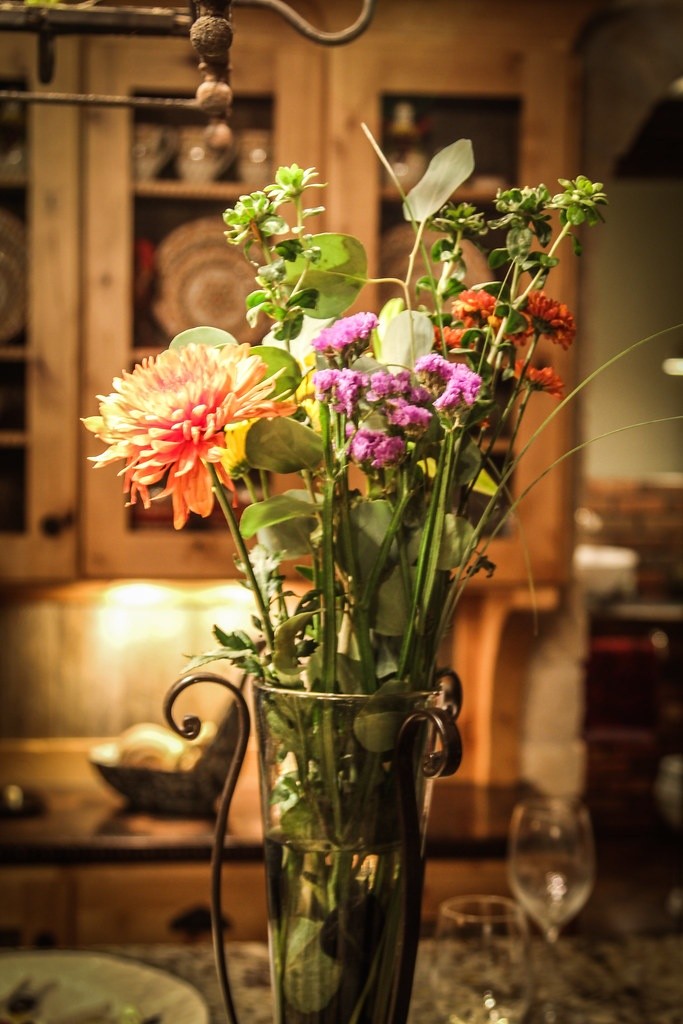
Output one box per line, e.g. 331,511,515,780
509,800,594,1024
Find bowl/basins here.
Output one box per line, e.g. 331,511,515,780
85,744,230,817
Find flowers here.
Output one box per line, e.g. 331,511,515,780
80,120,682,1024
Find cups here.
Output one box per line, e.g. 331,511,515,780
428,895,532,1024
572,545,637,597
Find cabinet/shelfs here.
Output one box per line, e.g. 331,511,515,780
0,0,619,594
0,859,520,950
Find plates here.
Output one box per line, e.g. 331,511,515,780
0,950,209,1024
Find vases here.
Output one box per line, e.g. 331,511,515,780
251,678,443,1024
